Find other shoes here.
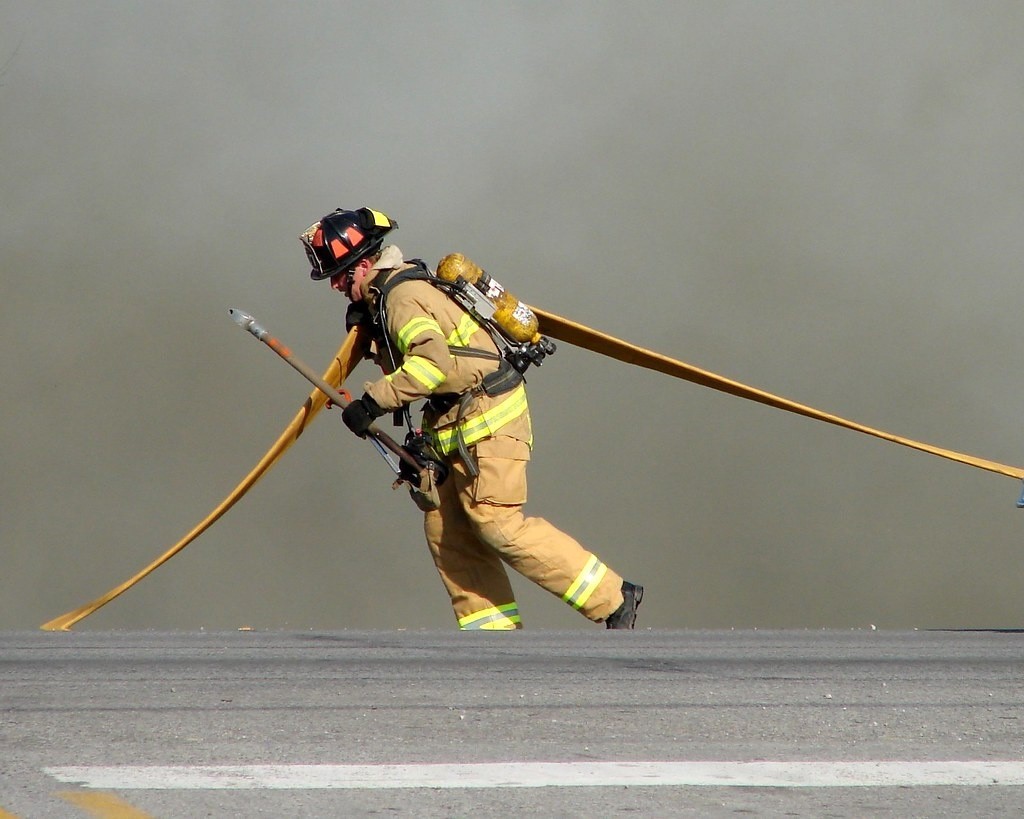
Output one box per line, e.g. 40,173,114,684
605,580,643,629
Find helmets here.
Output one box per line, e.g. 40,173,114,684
298,205,399,280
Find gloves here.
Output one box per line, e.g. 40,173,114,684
345,298,374,355
341,391,386,440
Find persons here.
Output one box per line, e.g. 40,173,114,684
301,206,644,631
